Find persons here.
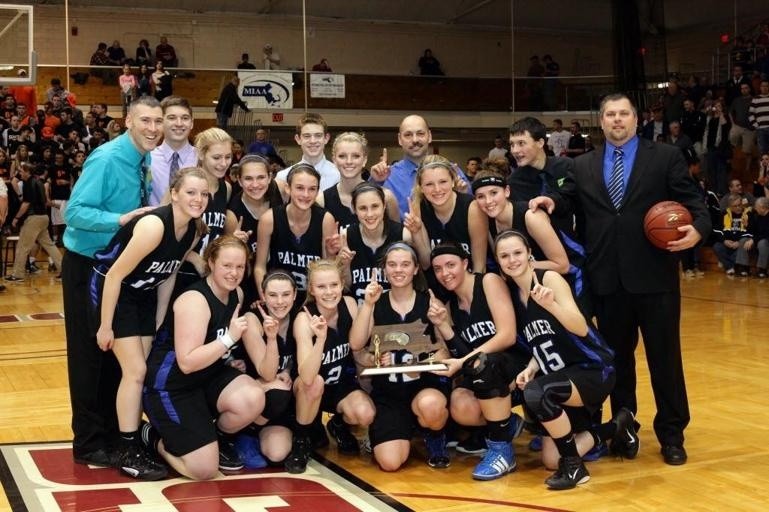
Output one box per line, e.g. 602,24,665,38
572,95,712,466
542,54,560,76
418,48,445,75
0,78,121,290
527,55,545,76
214,76,250,127
62,94,636,490
89,35,178,104
263,44,281,69
238,54,256,69
312,59,332,71
642,66,769,278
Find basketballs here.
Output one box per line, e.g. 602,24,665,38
644,201,693,250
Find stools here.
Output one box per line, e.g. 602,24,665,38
4,236,31,276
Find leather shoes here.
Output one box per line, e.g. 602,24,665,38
660,444,687,466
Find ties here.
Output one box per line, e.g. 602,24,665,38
538,173,551,196
169,151,179,190
606,146,624,210
139,158,150,208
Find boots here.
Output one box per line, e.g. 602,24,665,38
471,437,517,480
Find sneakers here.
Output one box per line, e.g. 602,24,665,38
528,436,542,451
427,457,450,469
74,418,328,483
610,406,639,459
544,454,590,490
0,261,62,293
582,440,609,462
325,419,362,456
455,434,486,455
444,436,459,450
685,266,766,280
502,414,524,440
